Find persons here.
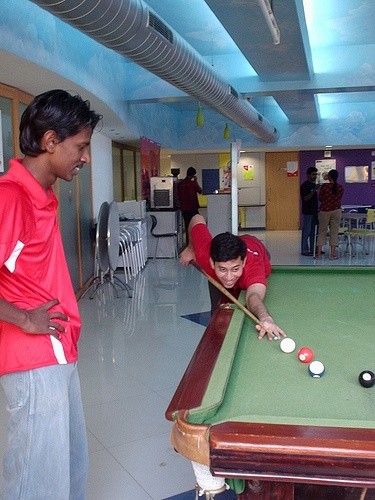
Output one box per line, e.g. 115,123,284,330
180,214,287,341
178,167,202,246
0,88,104,500
314,170,344,259
300,167,325,256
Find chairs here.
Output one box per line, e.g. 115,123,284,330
313,208,375,259
119,223,147,285
149,215,178,263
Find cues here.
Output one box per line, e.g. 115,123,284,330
189,259,278,341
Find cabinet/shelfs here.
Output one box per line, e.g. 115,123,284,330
148,211,183,258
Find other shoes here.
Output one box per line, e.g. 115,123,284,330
321,251,325,254
302,252,312,256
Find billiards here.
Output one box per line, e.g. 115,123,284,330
358,370,375,388
298,347,313,363
280,337,296,353
308,361,325,379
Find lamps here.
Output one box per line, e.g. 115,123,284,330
324,151,331,157
196,101,204,126
224,122,230,139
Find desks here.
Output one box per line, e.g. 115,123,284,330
77,200,134,305
239,204,266,230
341,205,372,213
341,213,368,260
202,193,231,237
166,265,375,500
